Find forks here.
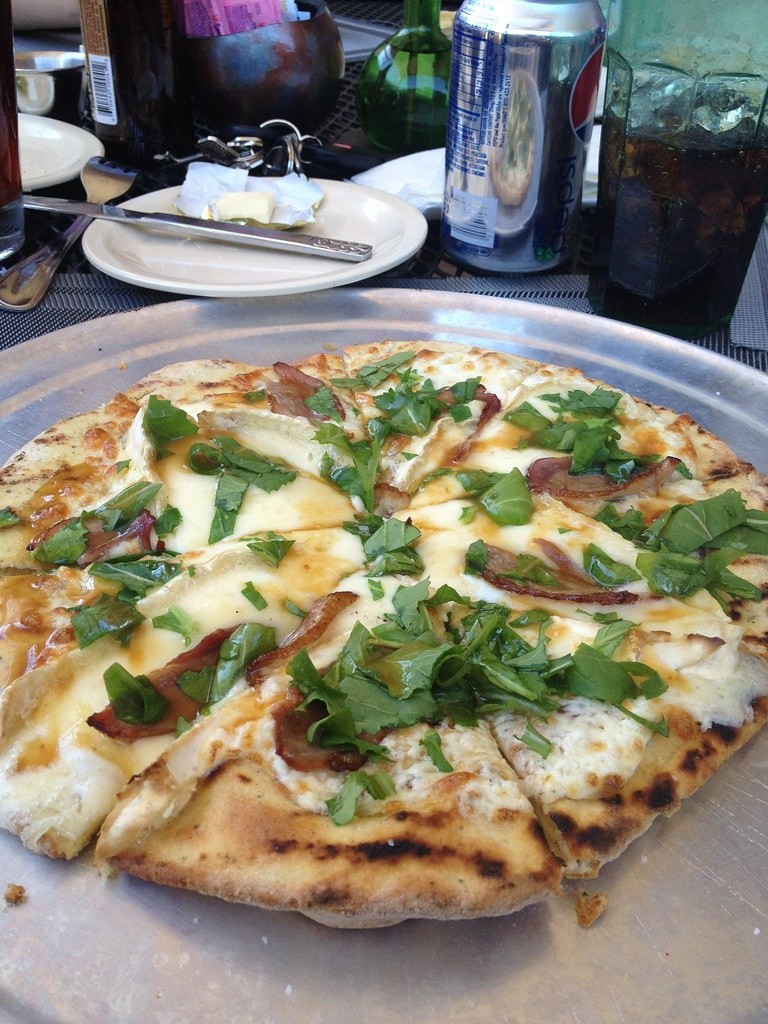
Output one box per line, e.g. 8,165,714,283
0,155,138,312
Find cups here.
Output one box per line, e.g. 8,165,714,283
584,0,768,338
13,48,88,125
0,0,25,261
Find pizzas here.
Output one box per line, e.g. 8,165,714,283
0,341,767,927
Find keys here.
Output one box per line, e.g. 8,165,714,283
154,116,305,176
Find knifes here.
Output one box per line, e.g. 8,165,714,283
17,191,373,263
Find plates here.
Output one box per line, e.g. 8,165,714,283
13,111,105,193
80,175,428,299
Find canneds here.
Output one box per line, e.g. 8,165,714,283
439,0,607,275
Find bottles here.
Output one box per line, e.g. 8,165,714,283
355,0,454,155
